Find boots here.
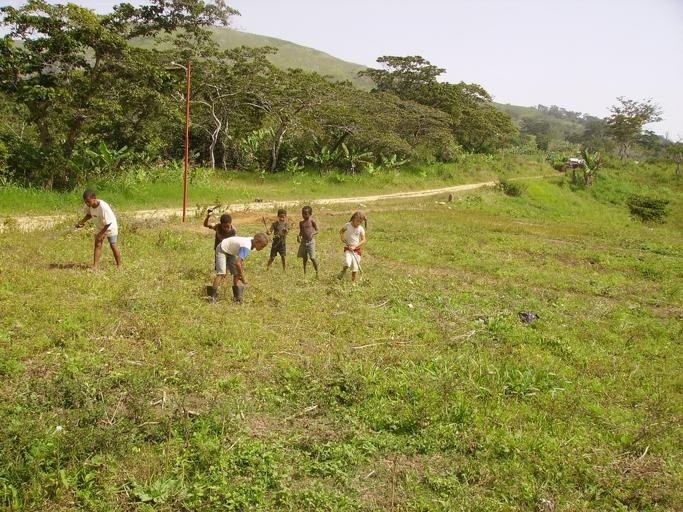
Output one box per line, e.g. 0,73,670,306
207,285,217,305
232,285,244,304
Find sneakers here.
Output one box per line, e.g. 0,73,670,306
336,274,343,280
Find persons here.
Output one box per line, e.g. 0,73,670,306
296,206,319,279
74,188,120,270
337,210,367,280
203,208,236,277
205,232,268,306
266,209,288,270
